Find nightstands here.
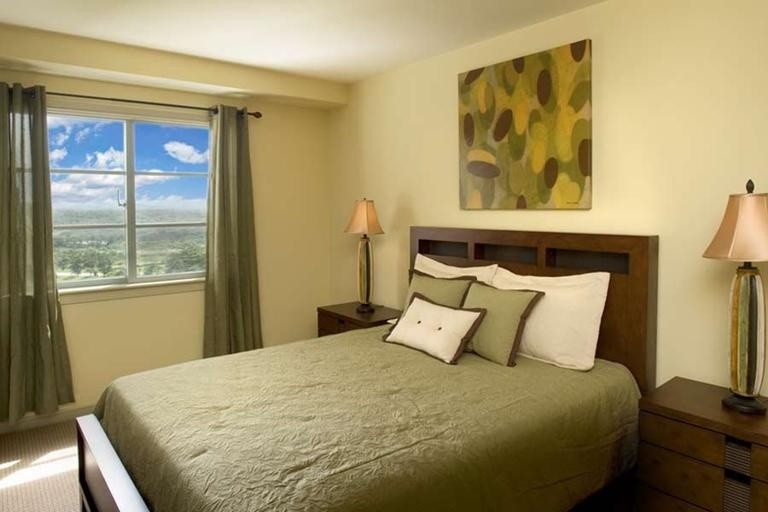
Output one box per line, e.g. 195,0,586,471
636,375,768,512
316,300,404,338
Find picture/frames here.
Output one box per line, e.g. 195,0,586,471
457,38,593,211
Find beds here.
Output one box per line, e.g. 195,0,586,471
74,224,660,512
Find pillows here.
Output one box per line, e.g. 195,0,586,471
389,268,477,332
386,252,498,324
382,291,487,365
490,266,611,371
459,280,546,368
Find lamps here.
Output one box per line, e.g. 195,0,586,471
344,197,385,313
702,178,768,415
